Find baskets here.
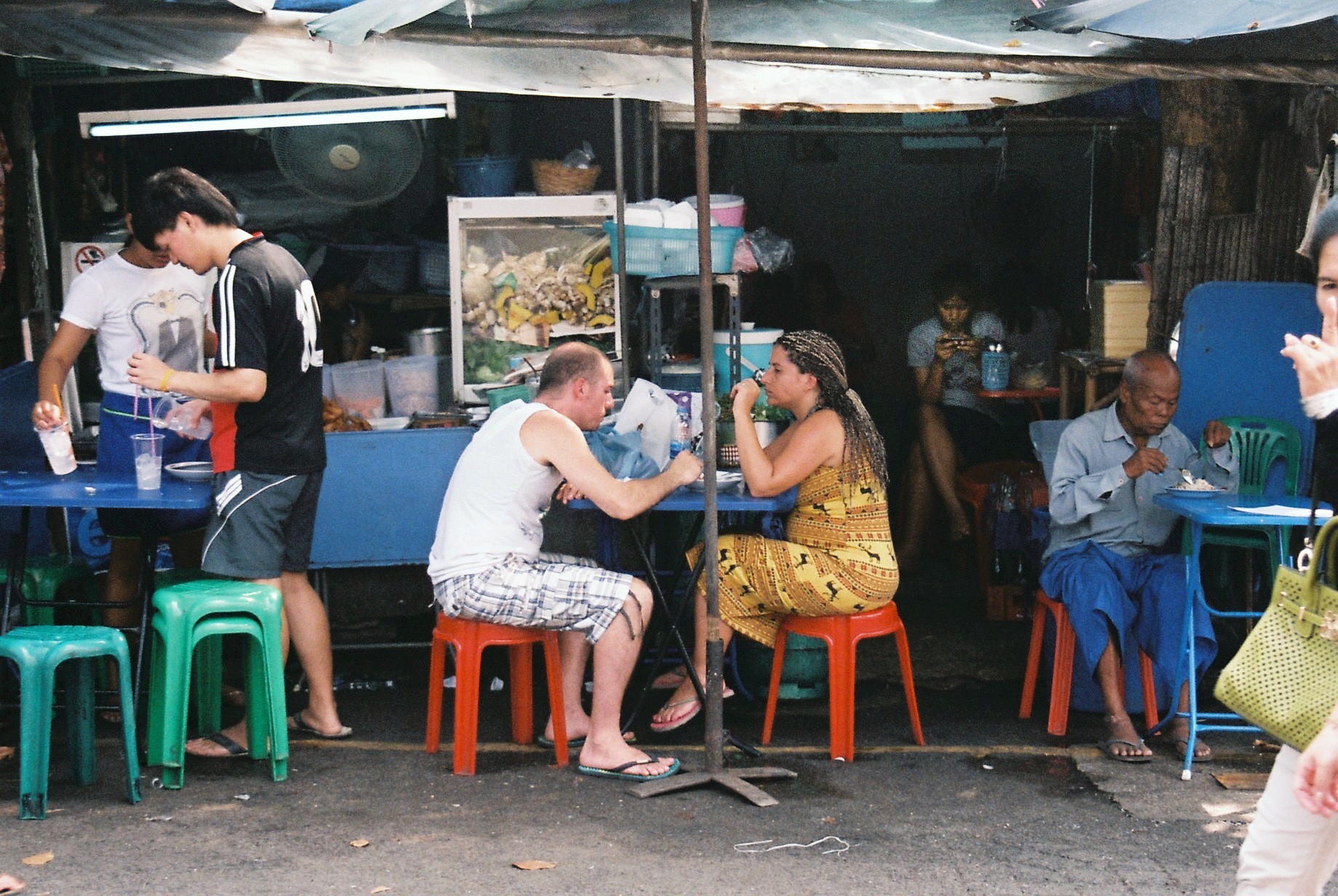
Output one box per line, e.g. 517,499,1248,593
603,220,744,277
531,160,601,194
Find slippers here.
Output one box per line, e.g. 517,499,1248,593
1164,737,1213,762
651,670,687,690
187,733,272,760
652,684,734,731
539,727,636,747
285,712,354,739
579,754,681,781
1097,738,1153,762
218,691,246,709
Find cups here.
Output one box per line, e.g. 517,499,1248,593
129,432,166,490
152,395,214,441
34,418,78,476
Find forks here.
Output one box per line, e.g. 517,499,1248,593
1166,462,1197,485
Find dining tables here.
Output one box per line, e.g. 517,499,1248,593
292,426,478,693
568,466,801,754
1140,494,1334,781
0,471,216,760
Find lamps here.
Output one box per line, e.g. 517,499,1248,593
78,92,456,138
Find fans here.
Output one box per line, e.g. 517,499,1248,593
270,83,422,206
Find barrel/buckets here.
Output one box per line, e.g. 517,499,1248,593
682,193,746,227
713,321,784,407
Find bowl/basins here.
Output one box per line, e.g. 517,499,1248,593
383,354,452,415
447,154,520,197
330,359,387,425
408,327,452,355
687,472,740,492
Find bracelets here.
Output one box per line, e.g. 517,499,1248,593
160,369,175,392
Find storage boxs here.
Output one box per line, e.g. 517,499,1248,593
416,239,450,297
1089,280,1151,360
337,243,416,296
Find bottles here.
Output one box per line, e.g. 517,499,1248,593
669,409,693,463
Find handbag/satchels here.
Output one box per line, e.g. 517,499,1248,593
584,379,678,480
1214,515,1338,753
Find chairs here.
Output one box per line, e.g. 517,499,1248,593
1180,418,1302,588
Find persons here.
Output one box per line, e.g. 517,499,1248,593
35,163,356,767
897,270,1007,567
645,332,899,729
1037,350,1241,763
1236,190,1338,896
428,342,704,780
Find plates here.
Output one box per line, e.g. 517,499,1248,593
1163,487,1226,498
366,416,410,431
164,461,214,482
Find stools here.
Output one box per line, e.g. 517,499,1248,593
957,459,1047,622
1019,588,1161,739
0,581,289,820
758,599,924,763
427,609,569,776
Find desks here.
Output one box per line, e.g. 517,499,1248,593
975,386,1059,458
1057,351,1126,420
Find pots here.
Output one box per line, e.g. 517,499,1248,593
715,419,778,468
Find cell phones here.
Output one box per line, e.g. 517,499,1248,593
945,337,965,344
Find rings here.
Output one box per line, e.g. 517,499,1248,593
1309,338,1321,347
